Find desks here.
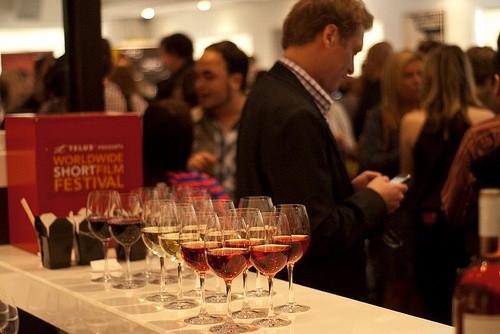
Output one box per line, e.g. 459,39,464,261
0,228,456,334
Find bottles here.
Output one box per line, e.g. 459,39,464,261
453,189,500,334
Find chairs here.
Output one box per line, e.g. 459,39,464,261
455,189,500,334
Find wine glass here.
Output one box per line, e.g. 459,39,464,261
141,200,180,303
107,192,147,290
268,204,310,314
87,191,121,283
204,216,250,334
199,200,239,304
128,185,211,217
237,196,277,298
180,211,221,326
246,212,292,328
158,204,198,311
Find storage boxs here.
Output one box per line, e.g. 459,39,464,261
6,111,142,256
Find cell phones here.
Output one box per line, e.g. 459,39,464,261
390,174,412,183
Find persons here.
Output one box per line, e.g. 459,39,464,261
0,35,267,210
236,0,408,304
331,33,500,325
